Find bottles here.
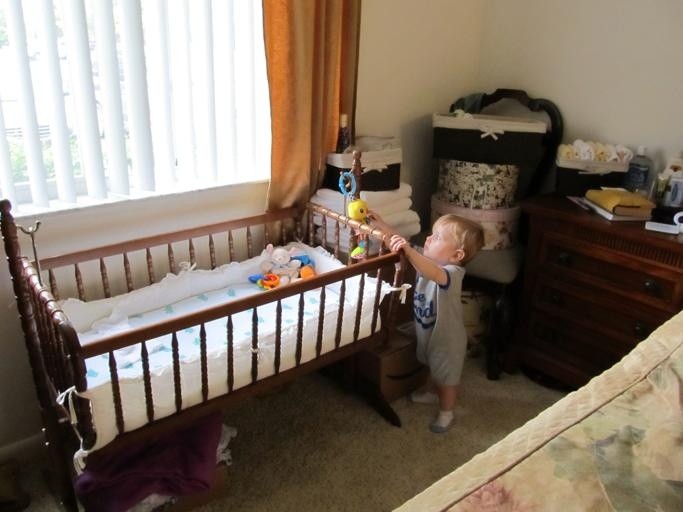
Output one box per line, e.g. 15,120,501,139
625,147,651,200
337,114,350,153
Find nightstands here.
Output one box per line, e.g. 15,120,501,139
498,187,683,399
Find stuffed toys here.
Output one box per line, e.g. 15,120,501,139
349,233,387,260
291,253,317,279
260,242,302,280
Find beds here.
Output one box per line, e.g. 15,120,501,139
391,309,682,511
0,193,437,511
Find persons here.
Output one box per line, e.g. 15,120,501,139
365,209,487,434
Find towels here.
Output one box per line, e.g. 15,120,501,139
301,182,430,258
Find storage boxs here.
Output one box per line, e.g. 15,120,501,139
320,131,406,194
345,339,446,406
424,95,555,249
548,141,630,195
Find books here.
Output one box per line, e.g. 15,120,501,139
576,198,592,213
579,196,652,223
643,203,682,235
584,187,656,218
